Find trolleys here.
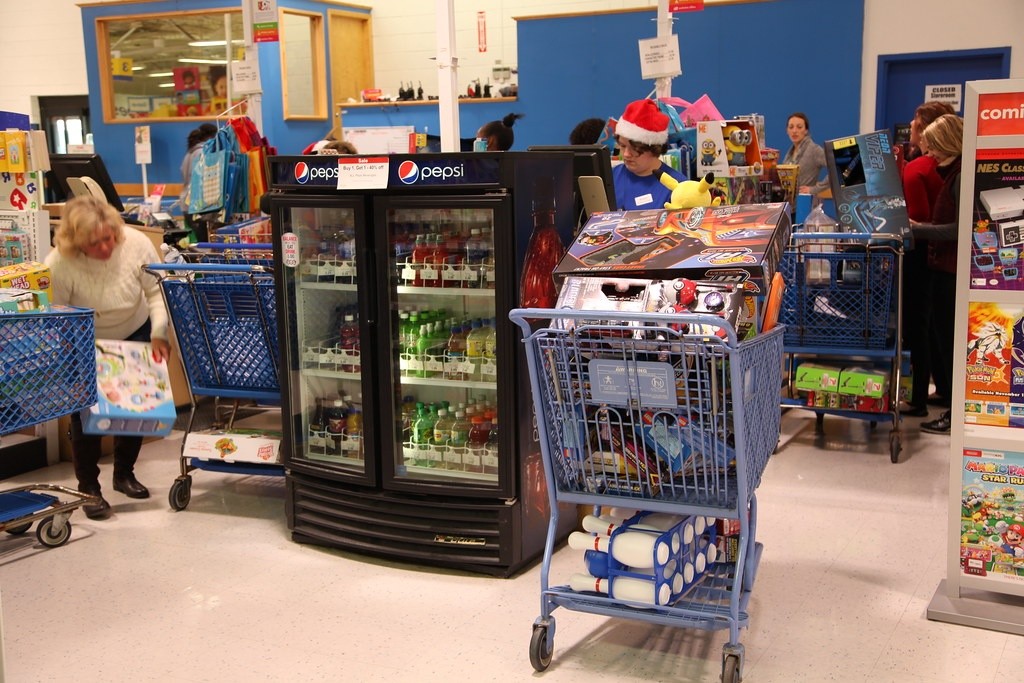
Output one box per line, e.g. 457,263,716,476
139,241,294,513
507,307,787,683
772,229,907,463
0,305,105,548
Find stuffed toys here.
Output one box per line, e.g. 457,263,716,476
652,169,722,209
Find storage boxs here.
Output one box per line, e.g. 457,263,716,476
175,92,200,104
899,377,913,402
0,261,178,434
112,58,132,75
825,127,913,248
839,368,889,397
183,409,283,465
177,104,202,116
552,120,792,563
796,363,841,393
808,391,888,413
217,214,275,261
173,68,200,89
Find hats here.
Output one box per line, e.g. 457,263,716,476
615,99,670,146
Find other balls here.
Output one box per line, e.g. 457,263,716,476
584,549,623,579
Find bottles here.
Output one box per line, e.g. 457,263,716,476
519,195,573,536
310,227,499,471
161,244,194,281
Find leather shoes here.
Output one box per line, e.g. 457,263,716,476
920,412,951,434
113,472,149,498
82,489,110,517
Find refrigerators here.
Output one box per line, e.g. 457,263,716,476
257,152,605,574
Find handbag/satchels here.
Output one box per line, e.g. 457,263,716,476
188,117,278,224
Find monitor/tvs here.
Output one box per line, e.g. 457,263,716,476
528,144,617,229
50,153,126,213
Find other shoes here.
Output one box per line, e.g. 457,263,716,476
899,402,928,417
928,392,951,406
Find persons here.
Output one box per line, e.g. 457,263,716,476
324,141,357,155
182,123,218,243
780,113,832,212
602,99,691,212
477,114,520,152
184,71,194,93
570,119,606,146
45,196,171,520
898,101,964,434
208,66,233,115
